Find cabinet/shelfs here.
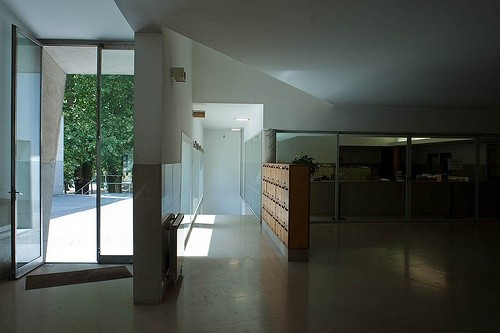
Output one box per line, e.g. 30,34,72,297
262,163,310,251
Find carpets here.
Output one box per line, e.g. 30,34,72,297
24,265,133,291
